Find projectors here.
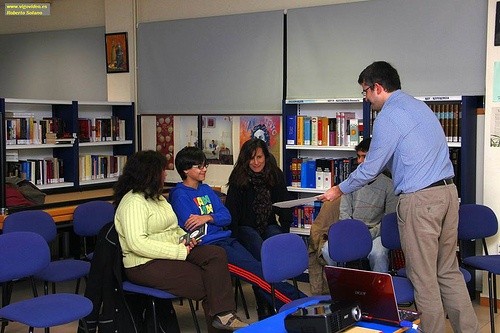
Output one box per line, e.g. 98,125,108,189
285,300,362,333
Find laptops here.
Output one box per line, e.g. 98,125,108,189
324,265,422,324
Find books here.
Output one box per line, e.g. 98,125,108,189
283,101,463,232
179,223,208,246
2,109,131,186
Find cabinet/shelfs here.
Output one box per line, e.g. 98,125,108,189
282,96,483,300
0,97,136,209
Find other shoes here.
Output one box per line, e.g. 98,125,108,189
212,313,249,330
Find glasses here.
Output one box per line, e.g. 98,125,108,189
361,82,381,96
193,164,209,169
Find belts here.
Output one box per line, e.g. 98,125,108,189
423,179,453,189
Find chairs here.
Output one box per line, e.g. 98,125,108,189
328,219,418,311
446,204,500,333
261,233,331,313
1,202,250,333
381,212,471,285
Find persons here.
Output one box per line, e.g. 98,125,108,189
167,146,310,313
111,150,251,333
224,139,298,320
321,138,401,274
316,60,481,333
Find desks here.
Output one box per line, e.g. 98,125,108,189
233,300,421,333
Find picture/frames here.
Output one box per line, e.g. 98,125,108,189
105,32,129,73
